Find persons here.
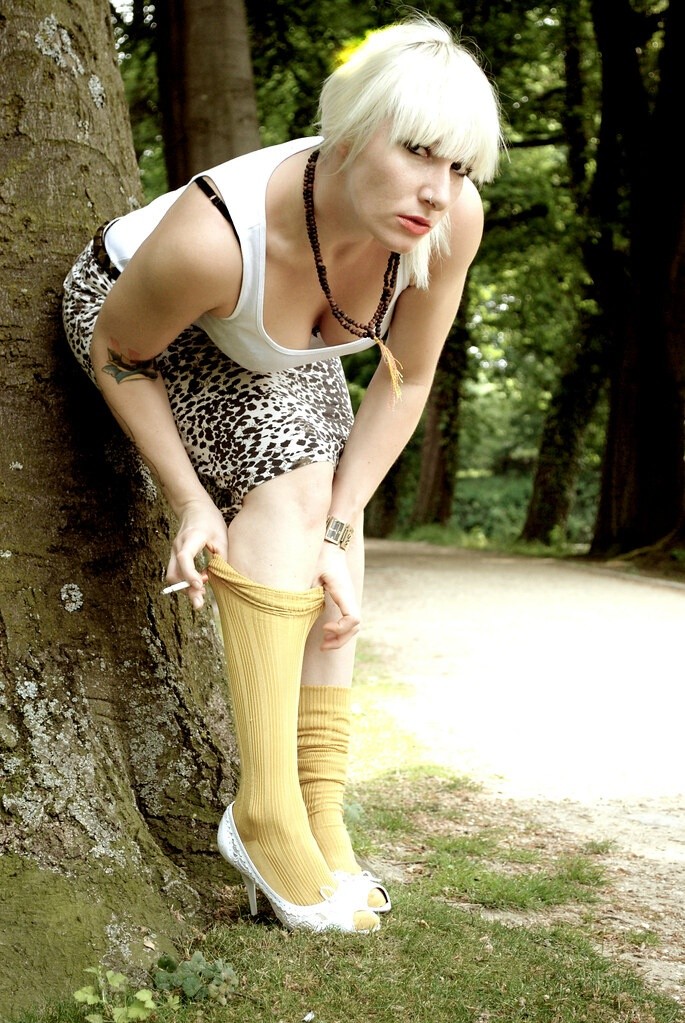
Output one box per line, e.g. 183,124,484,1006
60,16,501,937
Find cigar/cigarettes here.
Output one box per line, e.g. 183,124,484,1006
159,571,209,594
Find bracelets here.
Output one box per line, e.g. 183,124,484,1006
324,516,354,549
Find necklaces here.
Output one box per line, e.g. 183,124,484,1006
302,150,405,406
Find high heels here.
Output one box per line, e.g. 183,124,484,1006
216,801,381,934
332,870,391,912
